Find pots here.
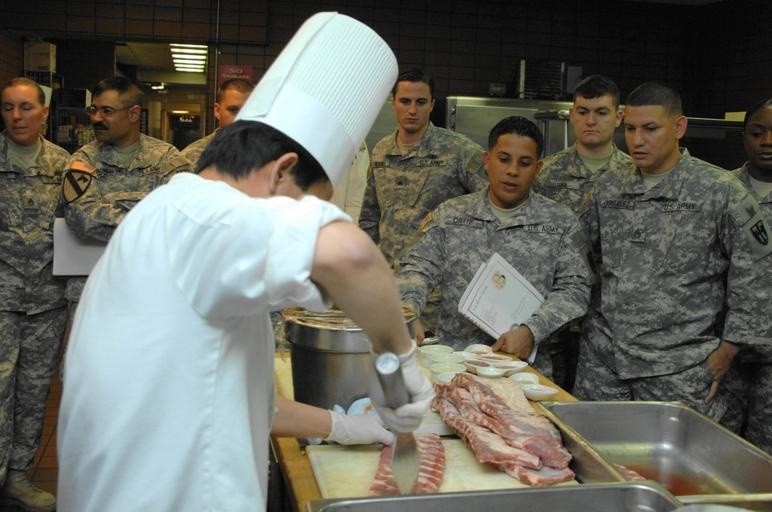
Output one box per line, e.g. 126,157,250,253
279,302,418,447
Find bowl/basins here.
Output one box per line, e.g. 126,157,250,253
420,340,558,401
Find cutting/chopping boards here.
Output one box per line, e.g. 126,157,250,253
305,436,580,497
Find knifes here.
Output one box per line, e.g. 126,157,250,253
373,350,422,492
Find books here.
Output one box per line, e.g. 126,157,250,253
458,253,549,364
51,216,107,278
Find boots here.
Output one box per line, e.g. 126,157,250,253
4,470,56,511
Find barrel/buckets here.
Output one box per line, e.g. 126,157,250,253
284,307,418,446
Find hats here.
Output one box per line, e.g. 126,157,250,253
232,11,398,193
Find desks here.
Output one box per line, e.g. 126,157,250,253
272,351,579,502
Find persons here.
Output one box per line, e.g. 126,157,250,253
55,117,439,512
529,76,635,394
729,100,772,449
566,82,772,423
397,116,594,385
0,78,74,511
60,76,192,383
181,77,253,174
372,68,491,337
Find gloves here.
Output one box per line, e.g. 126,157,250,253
370,339,437,434
324,408,393,446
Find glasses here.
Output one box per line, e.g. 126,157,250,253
85,106,134,114
